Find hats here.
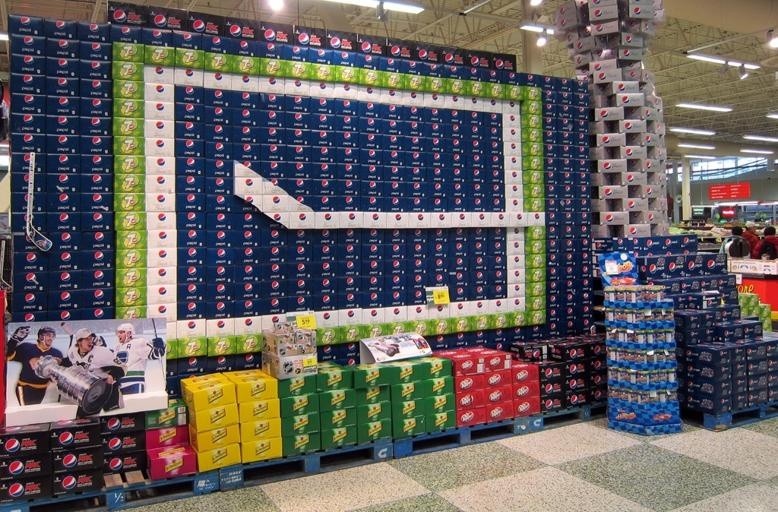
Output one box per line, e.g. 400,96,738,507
75,328,96,342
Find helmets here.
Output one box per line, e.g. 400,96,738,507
117,323,136,339
37,326,56,343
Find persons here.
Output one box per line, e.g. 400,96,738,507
4,325,65,407
719,226,750,259
752,226,778,261
93,323,165,395
50,329,127,419
742,223,761,259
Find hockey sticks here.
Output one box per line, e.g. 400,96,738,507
61,322,74,356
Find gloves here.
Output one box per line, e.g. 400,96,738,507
10,326,31,345
147,338,165,360
